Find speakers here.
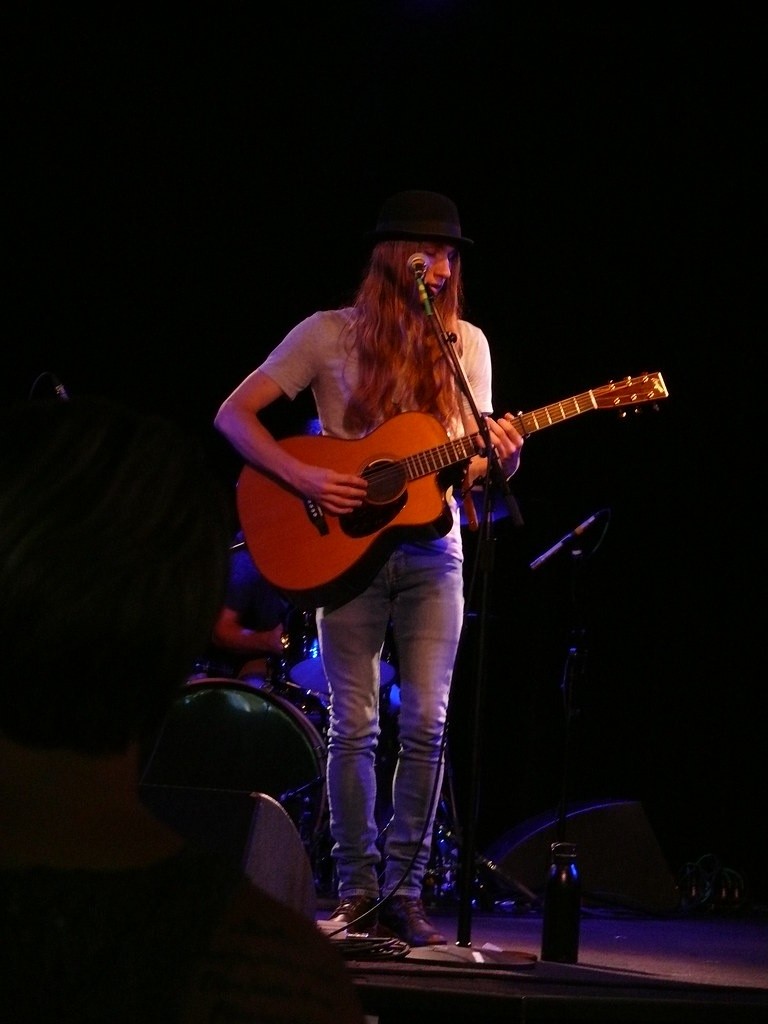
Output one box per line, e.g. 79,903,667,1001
482,800,684,918
52,783,317,937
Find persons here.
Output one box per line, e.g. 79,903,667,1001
213,190,526,946
213,531,290,688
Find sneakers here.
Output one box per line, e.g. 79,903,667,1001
375,895,448,947
317,895,379,938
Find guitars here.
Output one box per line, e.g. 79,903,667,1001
234,370,670,609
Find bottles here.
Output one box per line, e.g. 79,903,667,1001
542,842,579,963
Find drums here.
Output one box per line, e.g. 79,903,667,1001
142,677,327,855
188,661,321,730
284,605,399,696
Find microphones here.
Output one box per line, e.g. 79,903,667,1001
406,253,429,279
530,511,606,570
47,373,68,401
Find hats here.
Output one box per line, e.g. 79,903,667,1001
362,190,474,251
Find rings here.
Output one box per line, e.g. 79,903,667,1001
280,637,289,649
